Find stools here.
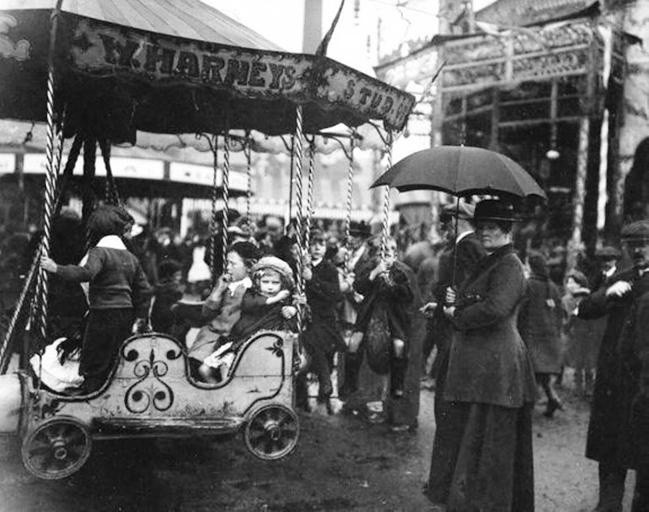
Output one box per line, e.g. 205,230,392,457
187,320,298,426
35,319,186,428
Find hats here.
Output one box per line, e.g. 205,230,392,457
459,200,525,222
620,221,648,242
443,202,476,218
350,222,373,236
251,256,293,277
595,247,620,260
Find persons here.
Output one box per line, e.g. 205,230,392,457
422,200,544,511
572,218,649,512
0,203,479,437
519,247,624,418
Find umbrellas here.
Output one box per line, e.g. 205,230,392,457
367,143,548,310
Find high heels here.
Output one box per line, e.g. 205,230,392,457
544,401,566,416
